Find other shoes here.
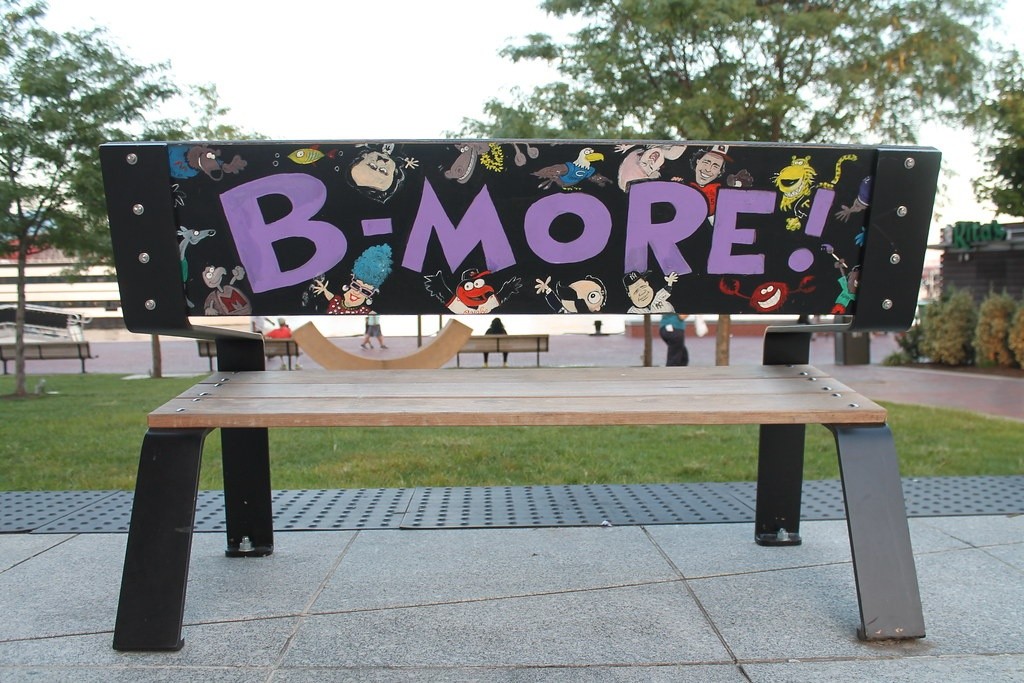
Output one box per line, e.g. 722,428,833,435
281,363,287,369
370,345,374,349
503,362,509,367
380,345,388,350
295,364,301,369
360,344,368,350
483,363,488,368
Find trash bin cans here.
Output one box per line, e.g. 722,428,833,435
834,315,871,366
918,302,932,324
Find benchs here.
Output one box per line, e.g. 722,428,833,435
452,333,549,369
0,341,98,374
97,136,943,650
196,337,300,371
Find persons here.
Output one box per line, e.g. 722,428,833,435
483,318,510,368
658,313,690,367
359,316,389,350
797,315,830,343
249,316,303,371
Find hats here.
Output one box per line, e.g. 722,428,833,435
278,318,285,325
